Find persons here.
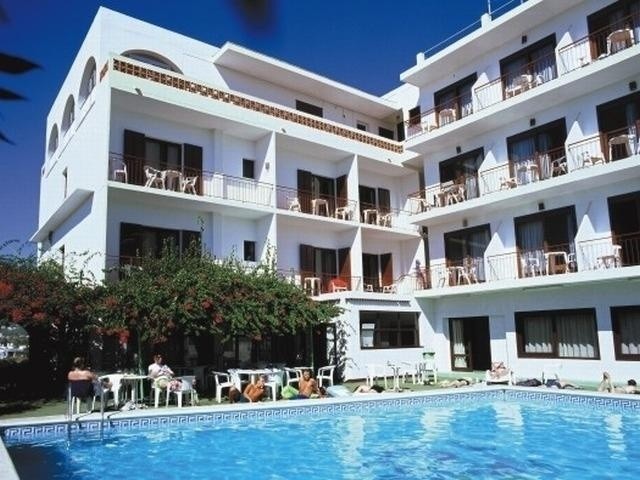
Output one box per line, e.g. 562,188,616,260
352,383,386,393
67,357,113,392
148,354,180,391
496,363,506,378
227,368,333,404
550,381,583,389
446,376,474,388
597,371,640,396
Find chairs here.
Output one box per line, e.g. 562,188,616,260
379,212,394,227
366,285,373,291
596,246,621,268
581,151,605,166
505,74,545,97
521,251,575,277
213,366,335,403
366,361,437,391
383,283,397,294
109,157,127,183
485,361,513,385
422,121,438,132
336,207,350,220
143,164,198,195
286,195,301,212
149,376,197,409
68,380,109,419
499,176,517,190
551,155,568,177
332,282,346,292
436,266,478,287
407,185,465,214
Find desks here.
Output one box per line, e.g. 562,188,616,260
517,165,538,185
606,29,635,55
312,199,329,217
304,277,321,296
608,135,634,161
439,109,456,126
91,375,149,411
362,209,378,225
